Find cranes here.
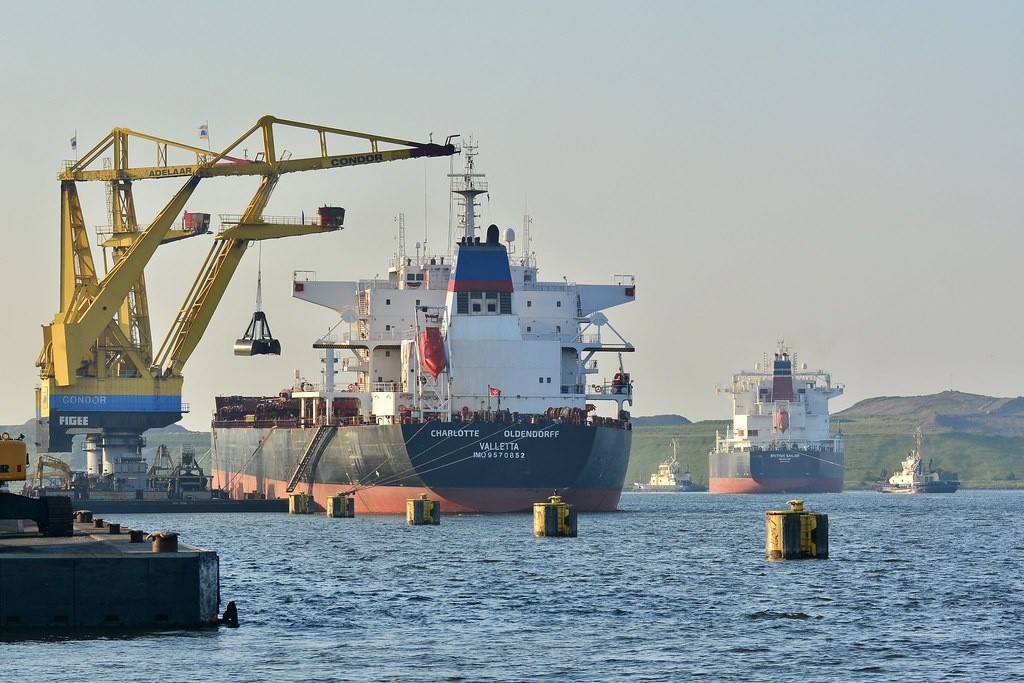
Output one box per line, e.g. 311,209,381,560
35,454,73,492
34,114,462,495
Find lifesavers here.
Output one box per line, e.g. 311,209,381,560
398,405,406,411
409,405,415,410
595,385,602,392
224,397,230,403
348,383,355,390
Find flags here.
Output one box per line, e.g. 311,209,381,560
489,386,500,397
197,123,208,140
69,136,77,151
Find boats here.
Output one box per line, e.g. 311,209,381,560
633,433,699,492
210,131,637,516
146,441,209,491
707,333,847,494
878,425,961,493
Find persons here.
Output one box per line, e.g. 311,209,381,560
23,482,32,497
